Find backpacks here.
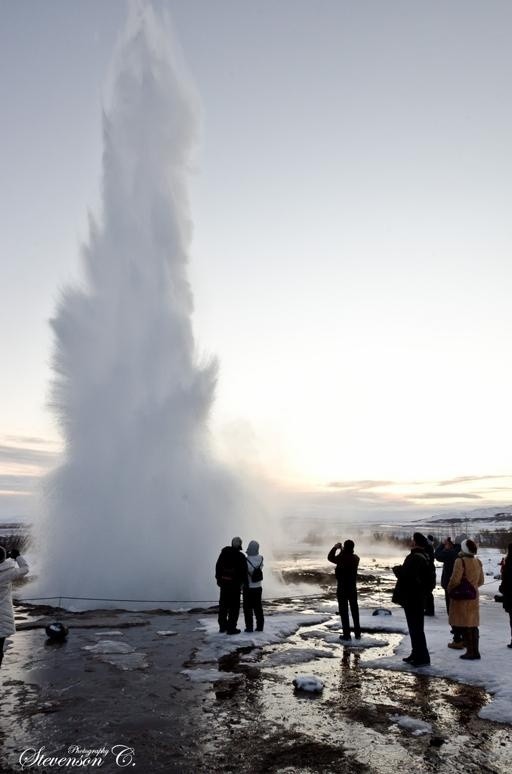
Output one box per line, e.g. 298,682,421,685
246,557,264,583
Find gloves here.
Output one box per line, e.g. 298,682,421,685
6,549,20,560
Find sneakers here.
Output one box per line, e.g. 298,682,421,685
424,610,434,616
340,634,361,641
218,627,264,634
448,630,481,660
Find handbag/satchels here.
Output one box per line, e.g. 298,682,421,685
449,579,477,601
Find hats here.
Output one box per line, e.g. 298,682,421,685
455,534,478,556
0,547,6,563
232,537,242,550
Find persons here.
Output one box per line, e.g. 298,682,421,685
0,545,30,668
424,535,436,616
391,532,432,667
493,543,512,648
243,540,264,632
327,539,362,641
435,533,468,649
215,536,249,635
497,557,505,575
446,538,484,660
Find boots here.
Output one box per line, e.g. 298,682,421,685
402,649,431,667
